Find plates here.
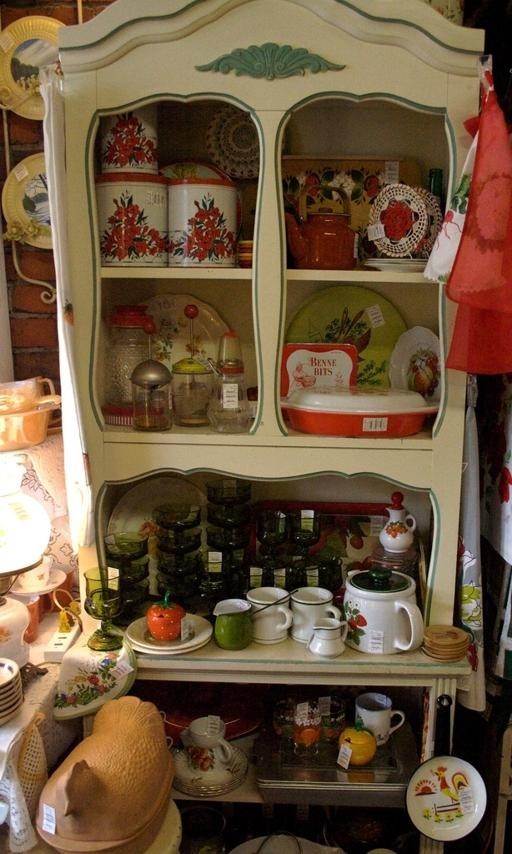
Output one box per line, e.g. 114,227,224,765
362,256,430,272
9,568,67,598
156,158,242,228
420,624,470,663
0,656,23,728
1,151,53,250
0,15,66,121
125,613,214,656
140,292,230,410
173,745,248,797
283,286,441,405
206,106,287,182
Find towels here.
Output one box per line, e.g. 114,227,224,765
53,620,138,721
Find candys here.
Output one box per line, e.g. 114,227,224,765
143,321,158,360
185,304,201,360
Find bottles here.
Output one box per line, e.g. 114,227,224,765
209,600,253,650
271,696,347,762
179,716,233,776
103,307,258,434
429,167,443,203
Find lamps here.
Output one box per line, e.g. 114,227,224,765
0,462,52,670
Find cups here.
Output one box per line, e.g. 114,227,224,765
355,692,405,747
245,587,348,658
379,491,417,554
16,555,53,590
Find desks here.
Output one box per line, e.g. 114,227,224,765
0,614,79,854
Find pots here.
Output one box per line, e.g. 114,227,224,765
405,695,489,843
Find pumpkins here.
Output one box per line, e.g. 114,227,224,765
148,590,185,641
338,719,378,766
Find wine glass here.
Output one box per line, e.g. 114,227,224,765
104,480,343,626
83,565,125,652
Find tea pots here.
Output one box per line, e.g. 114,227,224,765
343,567,425,656
285,184,361,270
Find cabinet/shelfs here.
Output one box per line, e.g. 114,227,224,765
0,3,84,305
57,0,485,854
